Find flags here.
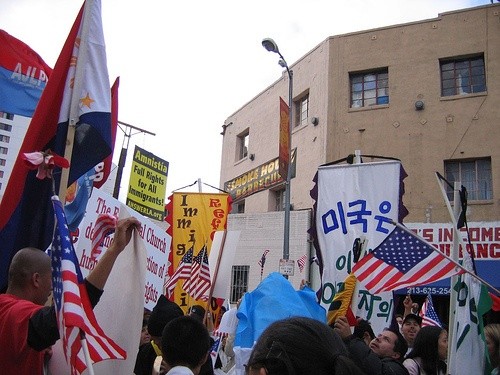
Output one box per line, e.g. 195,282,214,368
0,0,485,375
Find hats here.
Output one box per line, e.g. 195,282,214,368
147,295,184,337
401,314,421,327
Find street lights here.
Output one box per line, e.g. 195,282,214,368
260,37,296,281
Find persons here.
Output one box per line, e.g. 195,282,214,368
0,216,499,375
